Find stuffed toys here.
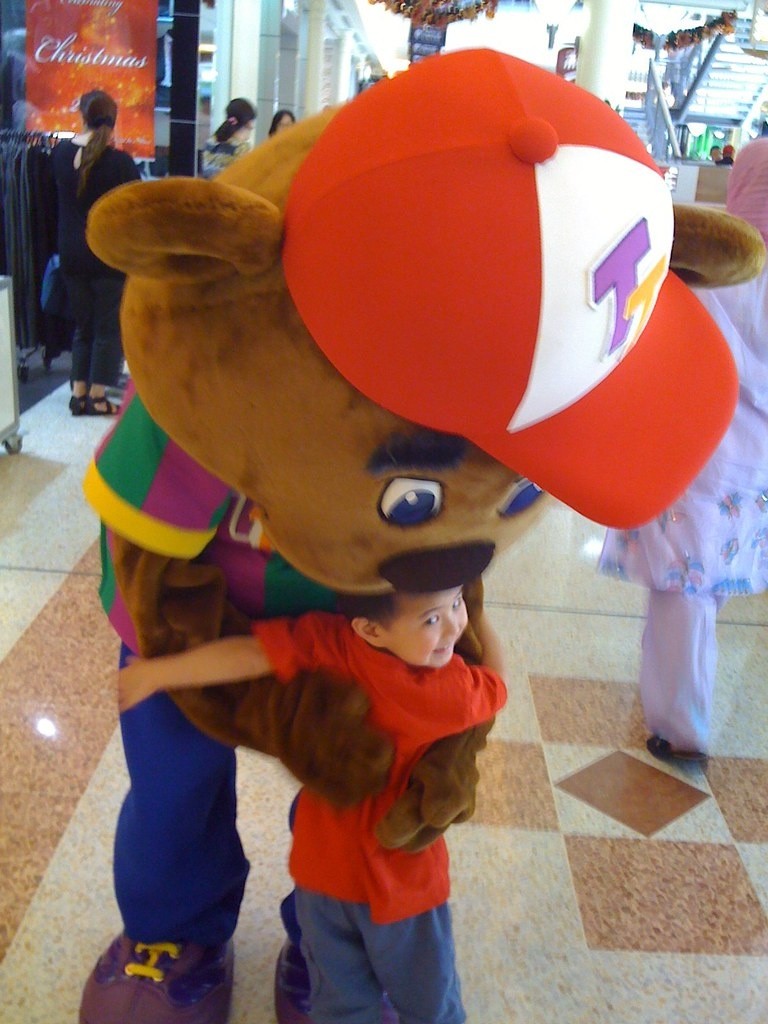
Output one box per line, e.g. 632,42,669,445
79,44,767,1024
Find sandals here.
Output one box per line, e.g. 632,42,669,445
85,395,121,414
69,394,90,416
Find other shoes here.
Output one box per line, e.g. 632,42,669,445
646,735,710,761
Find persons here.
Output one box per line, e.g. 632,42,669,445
596,133,767,768
269,110,296,137
106,584,510,1024
197,96,257,181
709,145,735,166
58,94,139,416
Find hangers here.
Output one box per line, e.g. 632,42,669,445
0,127,65,152
